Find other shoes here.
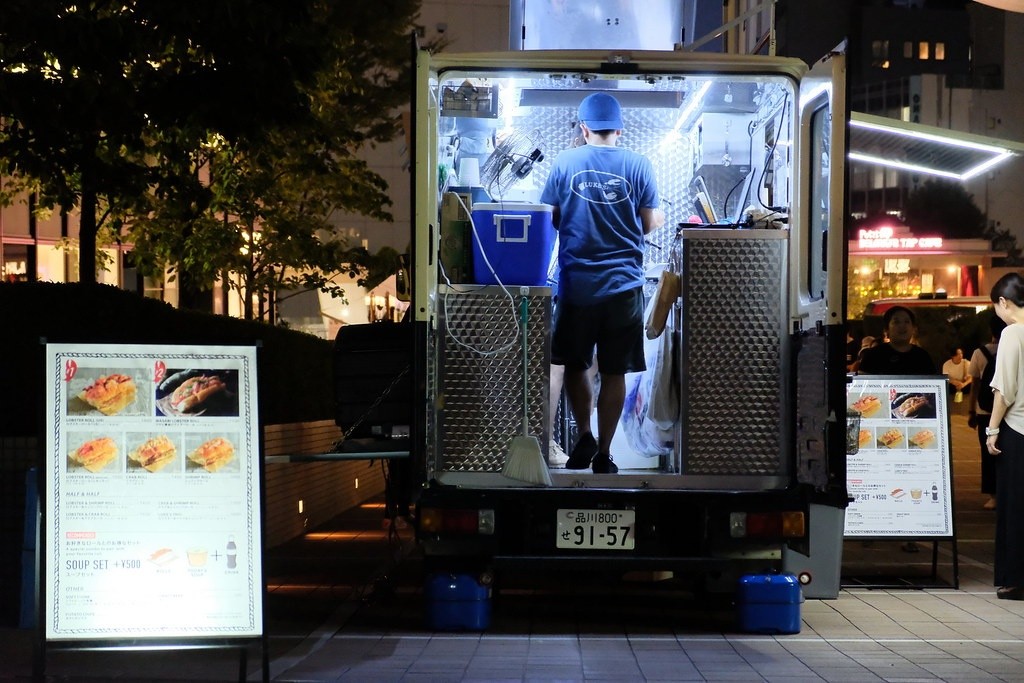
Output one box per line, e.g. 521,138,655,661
899,542,921,552
997,586,1024,600
983,496,996,510
565,431,599,469
592,455,619,474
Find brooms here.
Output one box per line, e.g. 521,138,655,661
501,295,554,488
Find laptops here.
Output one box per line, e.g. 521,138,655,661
678,167,757,229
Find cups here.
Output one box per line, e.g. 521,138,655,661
459,157,480,186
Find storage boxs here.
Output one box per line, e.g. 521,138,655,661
471,203,556,285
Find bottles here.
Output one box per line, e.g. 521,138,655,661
932,482,938,500
226,534,237,569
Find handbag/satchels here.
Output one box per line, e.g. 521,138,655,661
622,226,684,459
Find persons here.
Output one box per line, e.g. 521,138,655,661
985,272,1023,601
847,320,921,372
536,93,660,472
967,324,1006,509
859,306,941,376
943,348,972,394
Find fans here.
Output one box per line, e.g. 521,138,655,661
479,126,547,196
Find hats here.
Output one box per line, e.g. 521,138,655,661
577,92,624,131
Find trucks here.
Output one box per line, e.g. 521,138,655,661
408,37,855,622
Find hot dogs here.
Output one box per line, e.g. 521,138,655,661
899,396,926,417
169,376,226,412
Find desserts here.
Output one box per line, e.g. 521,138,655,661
68,437,118,473
127,434,177,473
186,437,234,474
850,395,881,417
877,429,904,449
858,429,872,447
908,429,934,449
77,374,137,415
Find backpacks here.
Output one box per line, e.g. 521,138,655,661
977,345,996,413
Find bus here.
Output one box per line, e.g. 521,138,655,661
863,291,997,367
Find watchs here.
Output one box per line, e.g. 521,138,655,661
986,427,999,436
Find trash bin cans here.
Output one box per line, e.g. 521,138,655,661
17,465,46,631
